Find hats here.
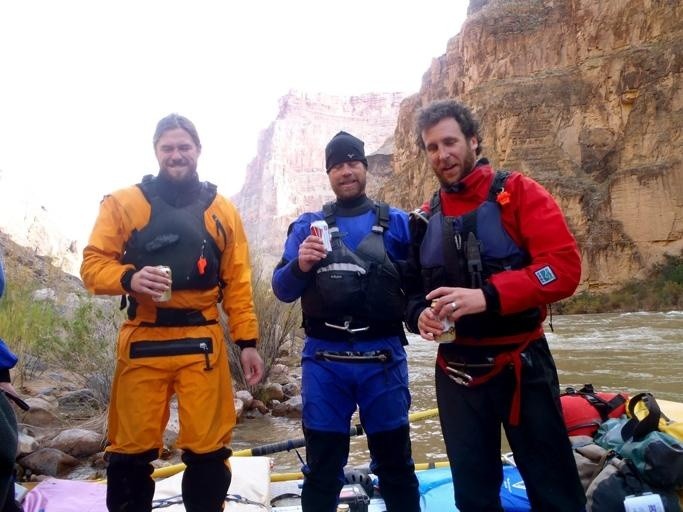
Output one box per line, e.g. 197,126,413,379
326,132,367,172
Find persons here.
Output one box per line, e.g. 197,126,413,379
272,132,423,511
81,115,264,512
406,100,586,511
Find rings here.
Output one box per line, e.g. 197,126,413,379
451,303,457,308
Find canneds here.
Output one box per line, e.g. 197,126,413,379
310,220,332,252
431,298,456,343
151,265,171,302
